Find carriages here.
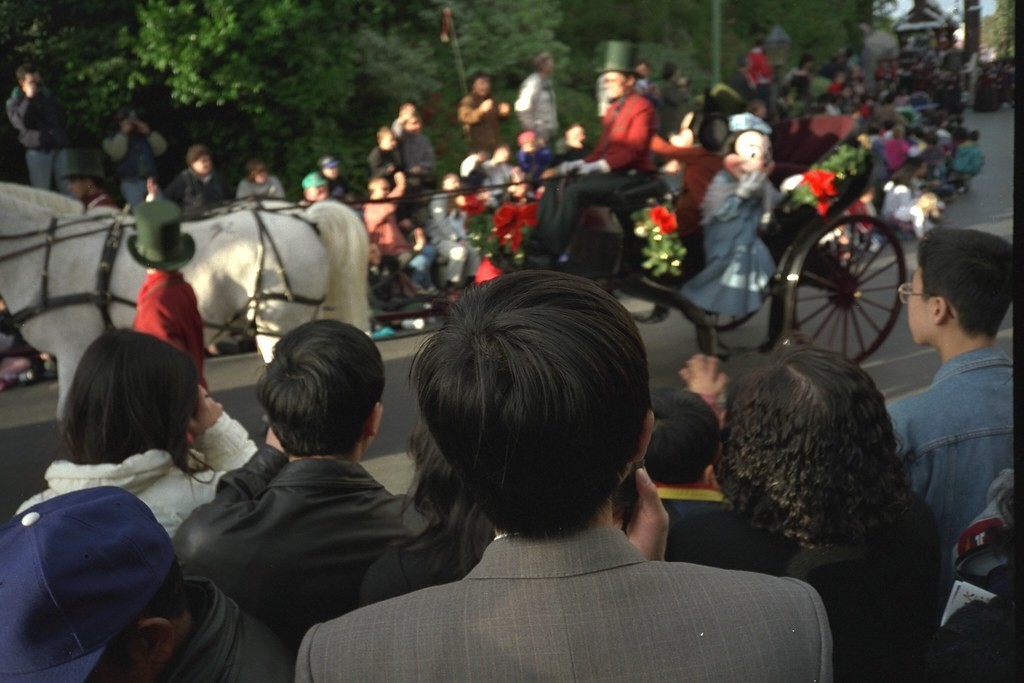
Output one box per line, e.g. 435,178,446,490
0,104,908,471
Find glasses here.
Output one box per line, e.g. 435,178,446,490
898,283,954,319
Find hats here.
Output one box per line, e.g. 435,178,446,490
301,171,330,189
126,199,195,270
594,41,644,80
58,145,106,180
0,486,176,683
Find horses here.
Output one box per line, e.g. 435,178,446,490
0,182,371,446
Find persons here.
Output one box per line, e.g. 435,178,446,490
0,1,1016,304
0,229,1024,682
124,197,215,391
